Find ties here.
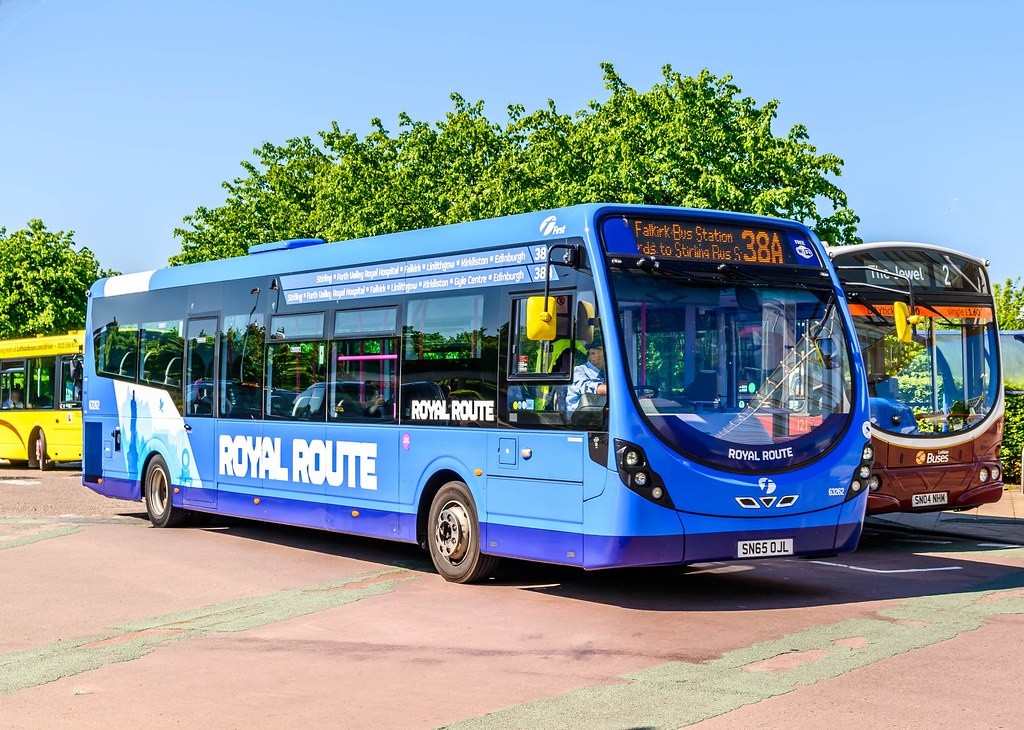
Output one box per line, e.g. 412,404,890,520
598,371,605,382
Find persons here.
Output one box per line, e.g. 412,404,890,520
565,337,610,423
209,352,390,423
534,339,589,411
3,389,24,410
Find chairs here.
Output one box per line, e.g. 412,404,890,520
115,347,450,423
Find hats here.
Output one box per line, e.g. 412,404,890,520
586,339,601,349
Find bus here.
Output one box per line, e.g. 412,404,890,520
0,329,89,470
79,202,875,583
487,242,1009,520
